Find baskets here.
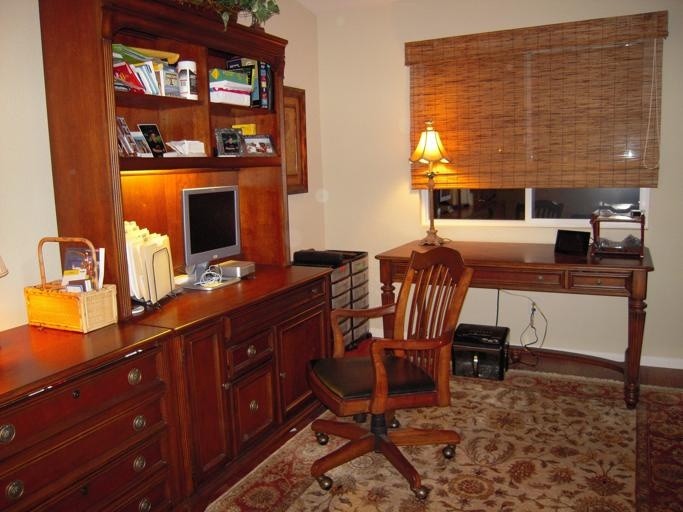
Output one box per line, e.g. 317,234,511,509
23,237,119,334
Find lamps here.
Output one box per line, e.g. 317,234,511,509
409,120,453,247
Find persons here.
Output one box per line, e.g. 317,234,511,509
115,116,275,158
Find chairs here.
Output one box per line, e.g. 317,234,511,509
304,246,474,499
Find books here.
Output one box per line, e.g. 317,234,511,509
110,42,273,108
60,220,177,307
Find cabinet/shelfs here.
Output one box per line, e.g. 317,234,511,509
173,317,235,508
0,329,189,512
221,270,333,479
38,0,288,175
330,253,369,350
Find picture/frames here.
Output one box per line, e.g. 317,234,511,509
240,135,274,157
215,128,242,158
283,86,308,194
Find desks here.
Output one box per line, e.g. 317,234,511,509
375,240,654,410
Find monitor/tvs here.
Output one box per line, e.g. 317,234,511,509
175,185,241,290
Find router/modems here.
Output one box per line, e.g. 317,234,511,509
214,260,255,278
